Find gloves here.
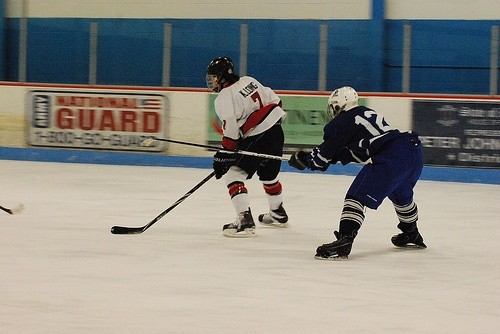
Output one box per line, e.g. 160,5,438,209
288,151,309,171
212,163,227,180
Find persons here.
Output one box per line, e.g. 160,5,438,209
287,86,423,257
205,56,288,232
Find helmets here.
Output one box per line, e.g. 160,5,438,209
328,86,359,111
205,56,240,82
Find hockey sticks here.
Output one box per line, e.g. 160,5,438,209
110,171,217,234
139,135,291,161
0,201,26,216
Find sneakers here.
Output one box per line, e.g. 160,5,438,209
223,207,257,237
391,223,427,249
259,202,289,227
314,230,358,262
